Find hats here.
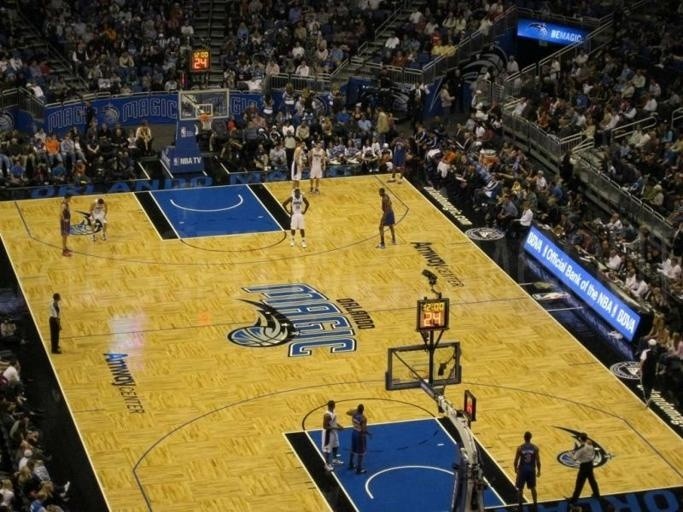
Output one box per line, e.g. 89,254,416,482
648,338,657,347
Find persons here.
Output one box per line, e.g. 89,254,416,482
474,198,682,408
282,188,310,248
376,188,397,249
321,399,345,472
566,432,599,504
57,193,75,257
0,315,71,512
1,1,681,197
48,293,64,356
88,198,109,241
513,431,542,512
347,403,374,473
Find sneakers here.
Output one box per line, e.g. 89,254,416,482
333,460,342,464
310,188,320,194
388,178,403,183
52,346,61,354
92,234,96,240
348,464,366,473
290,239,294,246
301,241,306,247
59,482,72,501
392,240,396,244
324,464,333,470
100,234,106,240
63,249,71,256
379,244,384,248
